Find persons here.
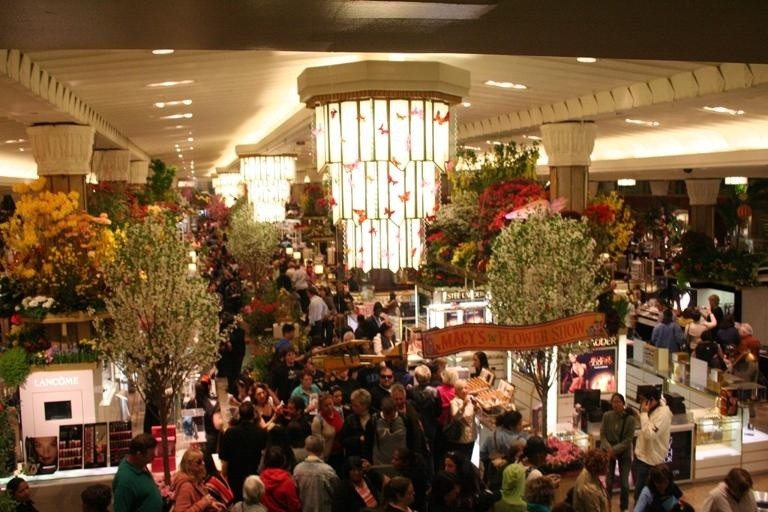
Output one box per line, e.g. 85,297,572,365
6,477,40,512
80,217,768,512
32,437,58,466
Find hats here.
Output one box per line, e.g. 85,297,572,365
525,436,555,454
734,321,754,336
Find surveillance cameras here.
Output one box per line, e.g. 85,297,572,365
683,167,693,174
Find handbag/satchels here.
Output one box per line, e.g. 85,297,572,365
680,338,688,352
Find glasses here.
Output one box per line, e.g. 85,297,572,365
379,374,394,380
